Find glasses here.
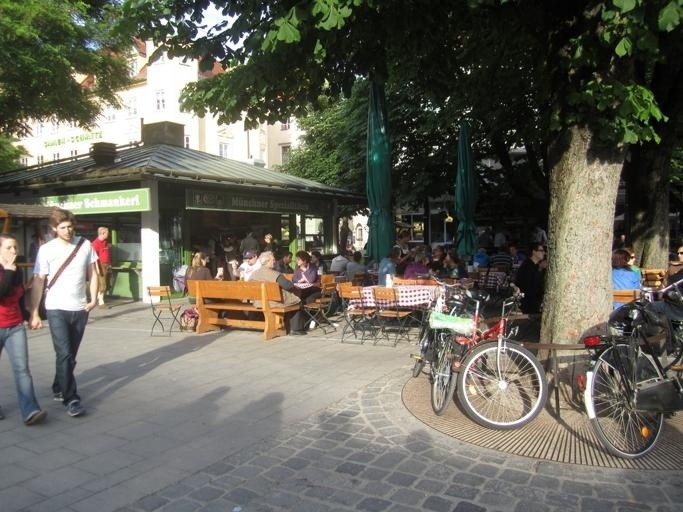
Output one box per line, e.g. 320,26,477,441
677,252,683,255
631,256,635,259
536,250,544,251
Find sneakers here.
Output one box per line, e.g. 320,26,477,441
25,410,48,425
54,392,64,401
68,400,84,417
100,304,112,309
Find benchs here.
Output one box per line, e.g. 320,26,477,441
186,278,293,343
632,266,667,287
613,289,643,304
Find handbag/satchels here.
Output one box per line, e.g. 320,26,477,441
38,287,48,320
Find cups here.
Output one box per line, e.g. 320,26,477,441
240,273,245,281
217,272,223,280
411,249,416,257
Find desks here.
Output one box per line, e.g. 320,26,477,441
15,262,34,285
339,277,445,305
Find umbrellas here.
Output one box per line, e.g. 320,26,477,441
454,115,481,257
357,65,399,259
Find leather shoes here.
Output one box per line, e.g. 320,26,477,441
290,330,306,335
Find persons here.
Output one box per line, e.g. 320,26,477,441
1,209,114,426
377,226,547,312
612,234,683,320
184,223,368,304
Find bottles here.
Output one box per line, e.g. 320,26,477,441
463,252,473,274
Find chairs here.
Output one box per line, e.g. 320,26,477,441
336,286,373,346
396,279,439,285
370,286,411,347
318,273,334,295
352,273,366,286
303,282,336,334
148,285,183,337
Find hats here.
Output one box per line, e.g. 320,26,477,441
243,250,256,258
